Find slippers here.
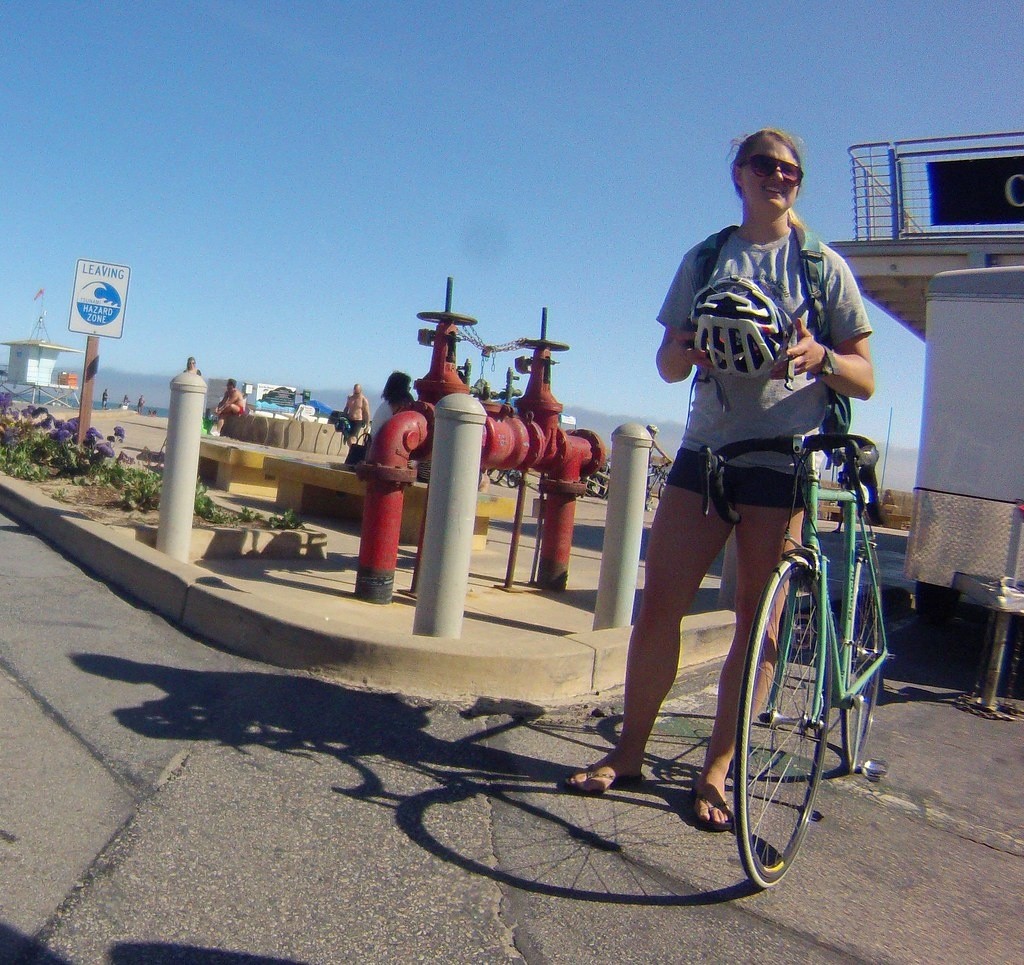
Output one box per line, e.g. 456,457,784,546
563,763,647,796
689,788,734,832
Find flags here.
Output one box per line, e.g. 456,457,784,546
34,288,45,301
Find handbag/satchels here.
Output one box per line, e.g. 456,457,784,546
345,431,372,464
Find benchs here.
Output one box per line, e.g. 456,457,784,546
262,455,515,550
199,436,284,498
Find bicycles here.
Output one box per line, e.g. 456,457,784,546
645,460,671,511
692,434,896,890
487,466,519,488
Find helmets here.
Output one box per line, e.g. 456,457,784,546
686,274,796,379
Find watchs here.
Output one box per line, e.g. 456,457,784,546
807,349,831,379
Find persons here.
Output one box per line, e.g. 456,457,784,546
646,424,673,512
138,395,146,414
210,378,246,437
389,390,490,494
123,395,131,406
102,389,108,409
365,371,412,467
151,411,157,417
341,383,370,448
183,356,202,377
566,126,875,836
832,470,848,533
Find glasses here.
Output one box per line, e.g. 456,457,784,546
741,154,805,186
